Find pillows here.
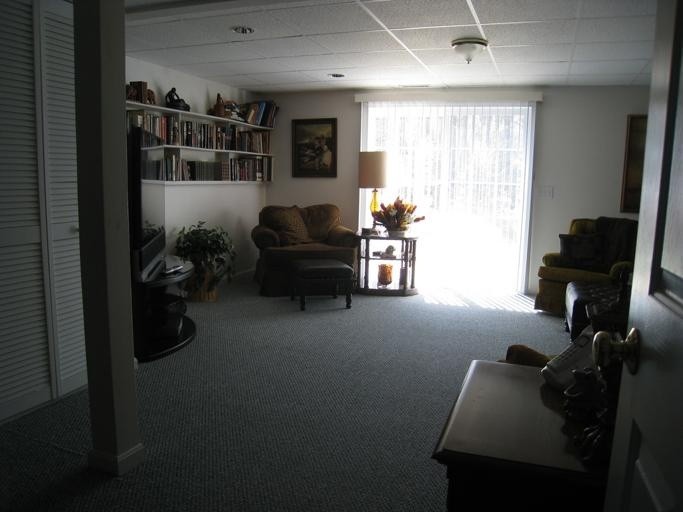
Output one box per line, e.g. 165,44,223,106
557,233,596,269
591,216,636,272
270,205,310,246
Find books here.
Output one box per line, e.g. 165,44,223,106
127,109,272,183
224,94,280,128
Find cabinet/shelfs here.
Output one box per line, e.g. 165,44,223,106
130,255,196,363
125,98,274,186
358,236,419,296
0,1,88,422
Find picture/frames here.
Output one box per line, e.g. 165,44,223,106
644,1,682,323
619,114,647,215
291,117,337,180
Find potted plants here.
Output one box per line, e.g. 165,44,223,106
173,220,237,301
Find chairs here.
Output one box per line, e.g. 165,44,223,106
533,213,637,318
250,203,359,298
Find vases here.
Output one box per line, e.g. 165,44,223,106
388,230,405,238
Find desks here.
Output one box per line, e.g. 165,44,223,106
433,359,605,511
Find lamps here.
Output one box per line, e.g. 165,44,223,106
451,37,488,64
358,150,385,227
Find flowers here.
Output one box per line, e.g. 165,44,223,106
372,197,426,230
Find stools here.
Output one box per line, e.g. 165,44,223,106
564,282,632,341
289,258,354,310
